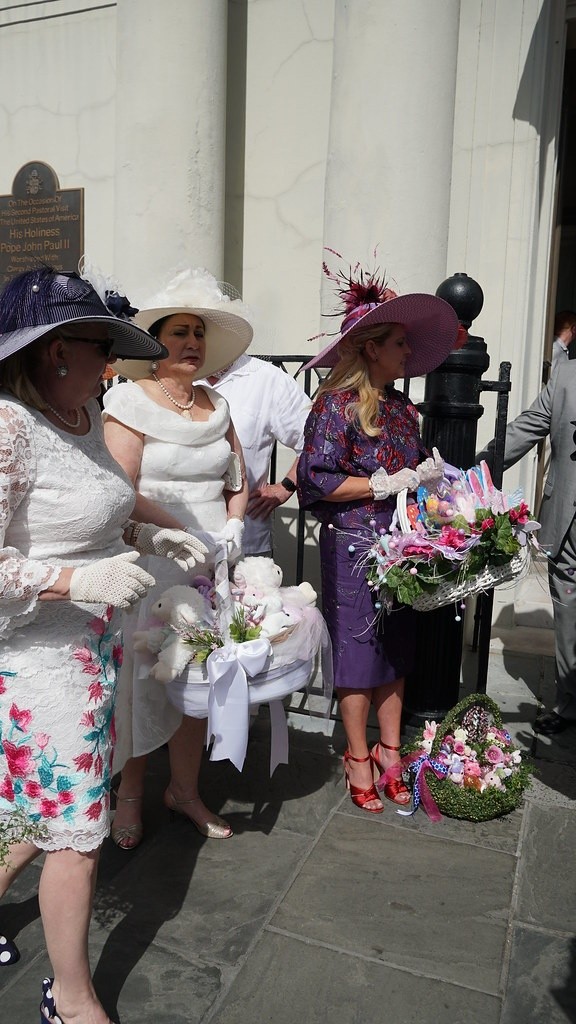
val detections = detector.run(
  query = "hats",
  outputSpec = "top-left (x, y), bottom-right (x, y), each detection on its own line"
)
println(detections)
top-left (0, 271), bottom-right (167, 361)
top-left (103, 285), bottom-right (254, 384)
top-left (299, 293), bottom-right (463, 378)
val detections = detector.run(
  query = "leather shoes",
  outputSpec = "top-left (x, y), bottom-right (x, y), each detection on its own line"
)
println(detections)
top-left (532, 710), bottom-right (570, 734)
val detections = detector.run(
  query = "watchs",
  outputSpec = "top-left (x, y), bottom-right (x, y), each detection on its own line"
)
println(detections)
top-left (281, 477), bottom-right (297, 492)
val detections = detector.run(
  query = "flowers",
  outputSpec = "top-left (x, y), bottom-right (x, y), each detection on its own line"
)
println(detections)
top-left (103, 252), bottom-right (254, 322)
top-left (422, 727), bottom-right (506, 764)
top-left (326, 492), bottom-right (576, 640)
top-left (170, 603), bottom-right (263, 663)
top-left (308, 242), bottom-right (400, 341)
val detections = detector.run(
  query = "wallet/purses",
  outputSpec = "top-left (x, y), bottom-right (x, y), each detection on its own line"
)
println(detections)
top-left (221, 451), bottom-right (243, 493)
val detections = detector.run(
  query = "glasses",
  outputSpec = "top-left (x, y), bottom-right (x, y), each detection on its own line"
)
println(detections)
top-left (60, 335), bottom-right (116, 358)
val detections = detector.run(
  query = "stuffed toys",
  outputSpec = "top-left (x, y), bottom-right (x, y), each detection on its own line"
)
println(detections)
top-left (131, 556), bottom-right (318, 685)
top-left (419, 721), bottom-right (522, 793)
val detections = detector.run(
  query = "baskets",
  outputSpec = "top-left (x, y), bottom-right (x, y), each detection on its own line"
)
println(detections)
top-left (160, 537), bottom-right (314, 716)
top-left (395, 483), bottom-right (528, 609)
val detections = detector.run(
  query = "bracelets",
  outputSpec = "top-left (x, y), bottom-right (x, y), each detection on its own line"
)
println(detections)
top-left (369, 477), bottom-right (373, 497)
top-left (126, 522), bottom-right (145, 547)
top-left (183, 526), bottom-right (187, 532)
top-left (230, 514), bottom-right (244, 522)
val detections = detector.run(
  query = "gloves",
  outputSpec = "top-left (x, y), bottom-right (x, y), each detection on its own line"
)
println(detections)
top-left (185, 518), bottom-right (243, 566)
top-left (137, 525), bottom-right (207, 574)
top-left (68, 549), bottom-right (155, 614)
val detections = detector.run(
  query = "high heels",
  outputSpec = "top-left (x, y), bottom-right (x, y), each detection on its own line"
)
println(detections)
top-left (112, 791), bottom-right (142, 848)
top-left (372, 738), bottom-right (410, 805)
top-left (342, 750), bottom-right (383, 813)
top-left (164, 787), bottom-right (232, 838)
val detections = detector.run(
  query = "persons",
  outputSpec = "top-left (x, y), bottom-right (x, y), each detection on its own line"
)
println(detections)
top-left (552, 309), bottom-right (576, 376)
top-left (298, 282), bottom-right (458, 813)
top-left (192, 355), bottom-right (314, 557)
top-left (101, 269), bottom-right (250, 850)
top-left (473, 359), bottom-right (576, 734)
top-left (0, 268), bottom-right (209, 1024)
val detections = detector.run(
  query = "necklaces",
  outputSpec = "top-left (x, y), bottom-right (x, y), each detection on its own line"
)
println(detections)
top-left (153, 373), bottom-right (195, 409)
top-left (44, 400), bottom-right (81, 428)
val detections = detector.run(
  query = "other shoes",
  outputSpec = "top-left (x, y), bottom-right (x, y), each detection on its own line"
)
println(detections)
top-left (39, 975), bottom-right (69, 1024)
top-left (0, 934), bottom-right (20, 966)
